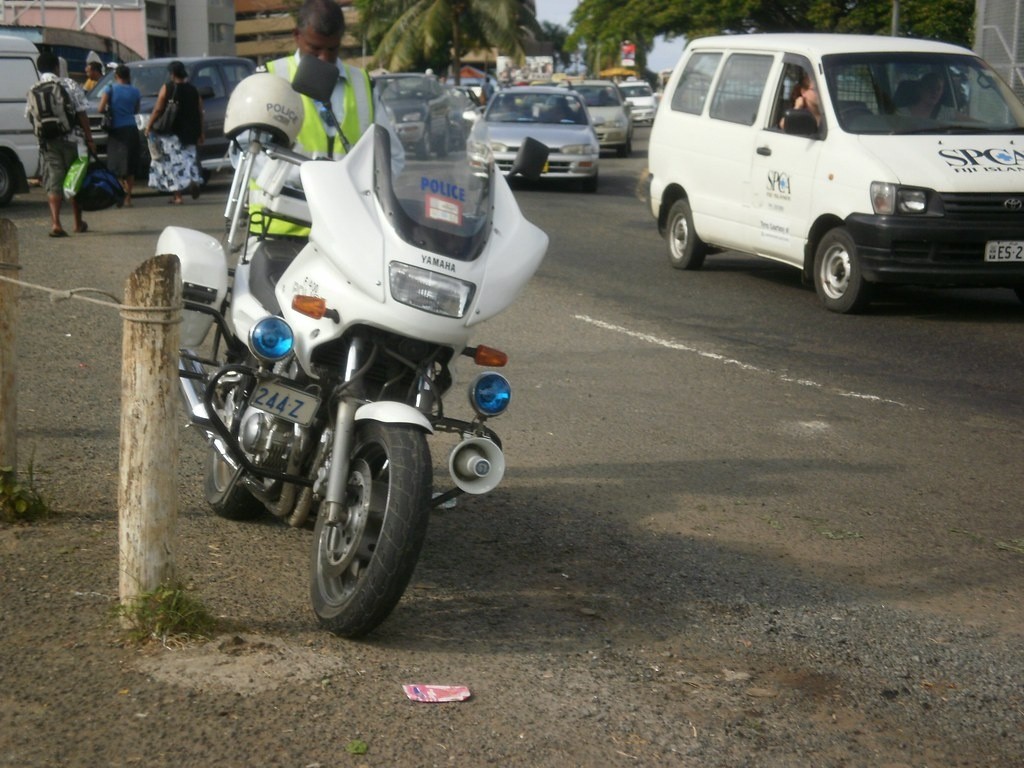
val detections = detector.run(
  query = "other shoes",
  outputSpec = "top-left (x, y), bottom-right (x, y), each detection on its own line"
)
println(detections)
top-left (190, 183), bottom-right (199, 200)
top-left (176, 199), bottom-right (183, 204)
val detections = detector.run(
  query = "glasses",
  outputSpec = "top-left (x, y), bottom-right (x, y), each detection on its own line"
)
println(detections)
top-left (808, 86), bottom-right (818, 93)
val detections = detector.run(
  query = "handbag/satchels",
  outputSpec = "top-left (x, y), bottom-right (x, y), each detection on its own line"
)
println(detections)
top-left (63, 143), bottom-right (89, 200)
top-left (75, 155), bottom-right (128, 210)
top-left (101, 85), bottom-right (114, 132)
top-left (151, 83), bottom-right (179, 132)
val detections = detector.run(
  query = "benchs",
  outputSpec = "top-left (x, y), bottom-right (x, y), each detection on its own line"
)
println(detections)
top-left (718, 99), bottom-right (875, 124)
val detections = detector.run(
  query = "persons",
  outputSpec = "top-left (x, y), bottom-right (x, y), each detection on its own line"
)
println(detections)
top-left (228, 0), bottom-right (405, 246)
top-left (83, 61), bottom-right (105, 95)
top-left (498, 63), bottom-right (512, 82)
top-left (894, 72), bottom-right (991, 123)
top-left (776, 75), bottom-right (821, 133)
top-left (482, 77), bottom-right (494, 102)
top-left (98, 65), bottom-right (141, 207)
top-left (23, 53), bottom-right (96, 237)
top-left (501, 97), bottom-right (525, 116)
top-left (144, 61), bottom-right (205, 204)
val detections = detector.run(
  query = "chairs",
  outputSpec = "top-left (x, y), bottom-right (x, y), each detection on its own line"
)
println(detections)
top-left (894, 80), bottom-right (922, 108)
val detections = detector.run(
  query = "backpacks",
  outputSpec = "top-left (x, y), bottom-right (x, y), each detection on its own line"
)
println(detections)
top-left (28, 77), bottom-right (76, 138)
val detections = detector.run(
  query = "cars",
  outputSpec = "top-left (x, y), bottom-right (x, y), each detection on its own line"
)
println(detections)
top-left (617, 82), bottom-right (659, 123)
top-left (369, 73), bottom-right (453, 160)
top-left (87, 56), bottom-right (259, 194)
top-left (442, 86), bottom-right (483, 143)
top-left (462, 86), bottom-right (600, 193)
top-left (557, 80), bottom-right (634, 158)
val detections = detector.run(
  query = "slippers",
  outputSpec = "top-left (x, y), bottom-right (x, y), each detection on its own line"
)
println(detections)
top-left (49, 228), bottom-right (68, 237)
top-left (73, 221), bottom-right (87, 231)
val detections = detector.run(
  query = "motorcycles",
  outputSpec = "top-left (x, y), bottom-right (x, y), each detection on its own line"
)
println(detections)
top-left (155, 78), bottom-right (551, 638)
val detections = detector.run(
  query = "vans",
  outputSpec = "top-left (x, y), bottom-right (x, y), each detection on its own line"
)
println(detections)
top-left (0, 35), bottom-right (47, 207)
top-left (648, 33), bottom-right (1024, 314)
